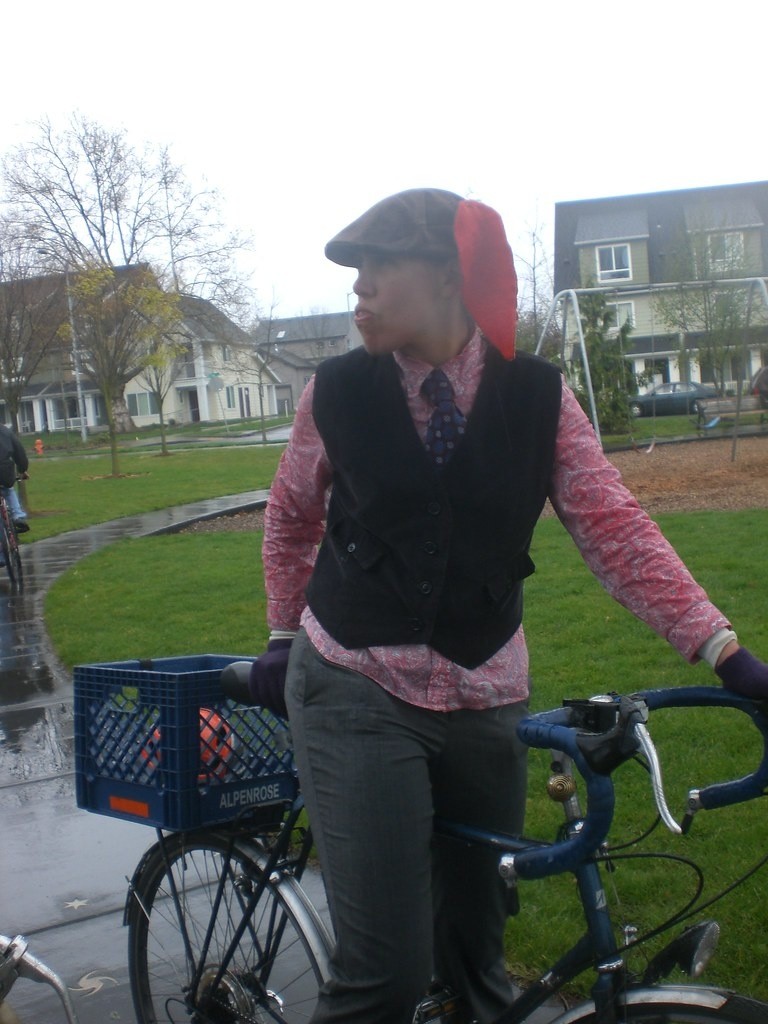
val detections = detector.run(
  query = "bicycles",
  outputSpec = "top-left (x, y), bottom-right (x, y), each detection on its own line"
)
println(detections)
top-left (118, 684), bottom-right (767, 1024)
top-left (0, 474), bottom-right (31, 581)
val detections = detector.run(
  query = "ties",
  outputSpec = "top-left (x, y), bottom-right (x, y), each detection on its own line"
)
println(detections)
top-left (421, 370), bottom-right (464, 465)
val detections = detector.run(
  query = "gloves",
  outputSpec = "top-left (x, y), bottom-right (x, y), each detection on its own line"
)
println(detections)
top-left (250, 639), bottom-right (293, 722)
top-left (715, 647), bottom-right (768, 708)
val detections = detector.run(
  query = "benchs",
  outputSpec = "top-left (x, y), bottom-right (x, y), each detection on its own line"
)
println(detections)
top-left (695, 394), bottom-right (768, 431)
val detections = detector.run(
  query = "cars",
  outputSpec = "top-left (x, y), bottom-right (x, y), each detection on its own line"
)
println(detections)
top-left (630, 380), bottom-right (734, 416)
top-left (750, 366), bottom-right (768, 409)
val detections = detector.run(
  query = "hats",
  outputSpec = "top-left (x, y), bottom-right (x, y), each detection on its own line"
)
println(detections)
top-left (325, 189), bottom-right (517, 360)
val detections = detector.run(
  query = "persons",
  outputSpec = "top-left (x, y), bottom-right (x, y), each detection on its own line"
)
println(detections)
top-left (0, 420), bottom-right (30, 532)
top-left (249, 187), bottom-right (767, 1024)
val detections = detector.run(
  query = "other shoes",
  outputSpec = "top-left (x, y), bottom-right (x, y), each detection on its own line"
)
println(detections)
top-left (13, 518), bottom-right (30, 531)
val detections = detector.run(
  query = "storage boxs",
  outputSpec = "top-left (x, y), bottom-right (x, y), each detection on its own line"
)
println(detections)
top-left (73, 653), bottom-right (300, 835)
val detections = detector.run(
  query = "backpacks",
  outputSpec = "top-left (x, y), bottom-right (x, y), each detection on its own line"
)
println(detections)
top-left (0, 431), bottom-right (15, 487)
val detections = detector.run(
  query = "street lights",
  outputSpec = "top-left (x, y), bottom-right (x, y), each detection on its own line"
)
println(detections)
top-left (36, 249), bottom-right (90, 449)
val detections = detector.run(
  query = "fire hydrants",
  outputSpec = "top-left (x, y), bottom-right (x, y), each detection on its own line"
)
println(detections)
top-left (34, 440), bottom-right (45, 455)
